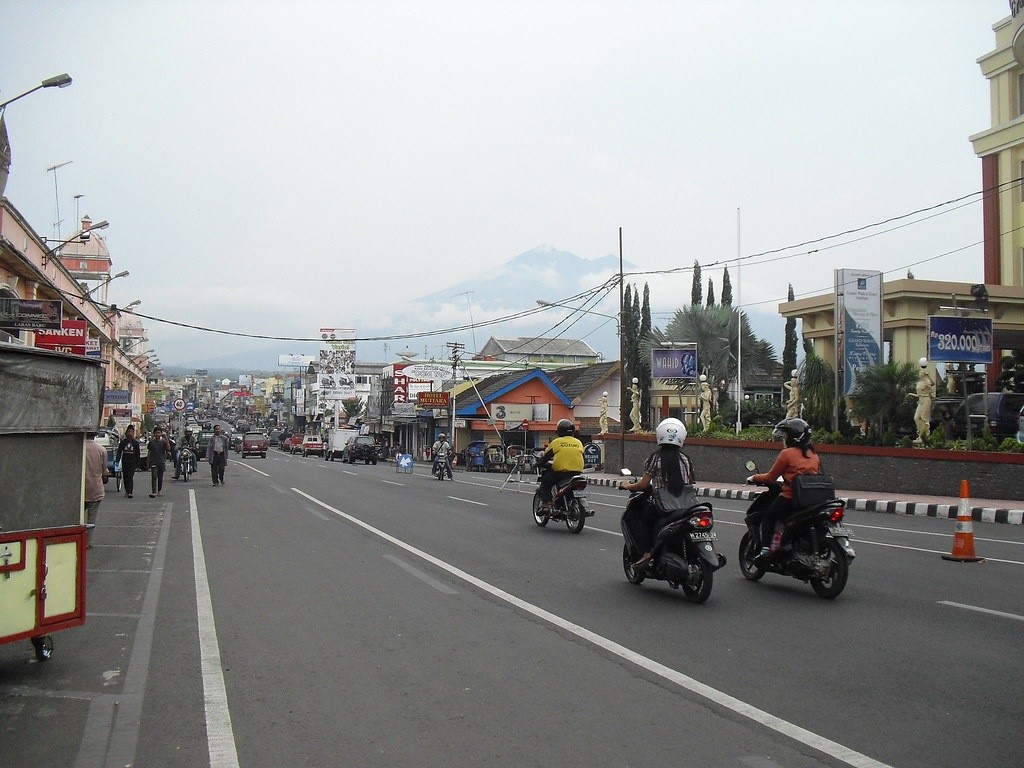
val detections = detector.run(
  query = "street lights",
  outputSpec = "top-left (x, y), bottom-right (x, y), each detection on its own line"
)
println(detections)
top-left (536, 299), bottom-right (625, 476)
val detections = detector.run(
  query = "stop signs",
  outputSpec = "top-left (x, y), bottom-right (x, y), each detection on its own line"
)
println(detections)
top-left (522, 418), bottom-right (530, 430)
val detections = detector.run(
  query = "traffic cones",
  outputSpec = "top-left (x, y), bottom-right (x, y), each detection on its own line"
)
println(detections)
top-left (941, 480), bottom-right (986, 563)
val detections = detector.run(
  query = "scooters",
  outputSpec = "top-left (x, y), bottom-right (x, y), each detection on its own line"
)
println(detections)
top-left (374, 441), bottom-right (403, 462)
top-left (617, 467), bottom-right (728, 605)
top-left (738, 459), bottom-right (858, 600)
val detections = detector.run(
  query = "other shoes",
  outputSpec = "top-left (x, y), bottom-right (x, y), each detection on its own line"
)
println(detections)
top-left (172, 476), bottom-right (179, 479)
top-left (149, 494), bottom-right (156, 498)
top-left (128, 494), bottom-right (133, 498)
top-left (221, 481), bottom-right (225, 484)
top-left (157, 491), bottom-right (163, 496)
top-left (212, 482), bottom-right (217, 486)
top-left (752, 550), bottom-right (771, 563)
top-left (630, 555), bottom-right (654, 569)
top-left (535, 506), bottom-right (549, 516)
top-left (193, 469), bottom-right (197, 472)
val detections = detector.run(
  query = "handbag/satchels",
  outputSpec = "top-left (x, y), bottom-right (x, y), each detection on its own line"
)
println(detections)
top-left (655, 485), bottom-right (701, 513)
top-left (431, 454), bottom-right (437, 461)
top-left (791, 474), bottom-right (836, 509)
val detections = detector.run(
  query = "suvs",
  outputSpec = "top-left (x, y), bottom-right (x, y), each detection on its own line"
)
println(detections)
top-left (930, 391), bottom-right (1024, 449)
top-left (341, 435), bottom-right (378, 465)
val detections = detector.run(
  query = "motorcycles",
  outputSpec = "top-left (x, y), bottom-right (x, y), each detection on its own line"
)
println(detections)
top-left (431, 447), bottom-right (452, 481)
top-left (531, 453), bottom-right (596, 535)
top-left (176, 441), bottom-right (201, 483)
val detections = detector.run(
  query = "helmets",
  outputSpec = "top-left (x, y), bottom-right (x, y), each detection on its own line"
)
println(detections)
top-left (772, 417), bottom-right (811, 446)
top-left (184, 427), bottom-right (193, 436)
top-left (655, 417), bottom-right (687, 448)
top-left (557, 419), bottom-right (575, 437)
top-left (438, 433), bottom-right (446, 438)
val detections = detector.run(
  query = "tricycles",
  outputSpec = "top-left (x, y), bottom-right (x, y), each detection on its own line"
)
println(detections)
top-left (94, 429), bottom-right (124, 492)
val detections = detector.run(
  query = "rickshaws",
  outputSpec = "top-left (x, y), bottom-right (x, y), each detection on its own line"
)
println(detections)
top-left (462, 439), bottom-right (549, 474)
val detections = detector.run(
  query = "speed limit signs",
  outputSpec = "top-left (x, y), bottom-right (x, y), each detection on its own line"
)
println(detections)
top-left (174, 398), bottom-right (186, 410)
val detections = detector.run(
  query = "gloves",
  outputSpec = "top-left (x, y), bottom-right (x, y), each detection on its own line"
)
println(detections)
top-left (746, 475), bottom-right (754, 484)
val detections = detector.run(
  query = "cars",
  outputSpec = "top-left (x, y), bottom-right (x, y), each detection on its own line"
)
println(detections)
top-left (281, 438), bottom-right (291, 452)
top-left (135, 405), bottom-right (296, 472)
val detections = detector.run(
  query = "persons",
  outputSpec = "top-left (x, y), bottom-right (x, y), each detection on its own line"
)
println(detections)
top-left (622, 418), bottom-right (693, 566)
top-left (206, 425), bottom-right (228, 486)
top-left (597, 397), bottom-right (609, 433)
top-left (908, 369), bottom-right (935, 443)
top-left (431, 433), bottom-right (455, 481)
top-left (84, 433), bottom-right (108, 550)
top-left (746, 418), bottom-right (820, 563)
top-left (172, 426), bottom-right (198, 479)
top-left (783, 378), bottom-right (799, 418)
top-left (699, 382), bottom-right (712, 432)
top-left (146, 427), bottom-right (168, 497)
top-left (534, 419), bottom-right (584, 516)
top-left (627, 384), bottom-right (641, 431)
top-left (116, 425), bottom-right (140, 498)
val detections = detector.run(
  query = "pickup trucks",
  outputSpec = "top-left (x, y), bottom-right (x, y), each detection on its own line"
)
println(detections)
top-left (300, 434), bottom-right (324, 457)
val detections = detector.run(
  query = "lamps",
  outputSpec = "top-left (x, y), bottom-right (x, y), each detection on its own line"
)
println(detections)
top-left (128, 349), bottom-right (155, 366)
top-left (80, 270), bottom-right (129, 307)
top-left (0, 73), bottom-right (72, 108)
top-left (119, 338), bottom-right (149, 360)
top-left (138, 358), bottom-right (163, 377)
top-left (103, 300), bottom-right (141, 328)
top-left (133, 354), bottom-right (156, 371)
top-left (42, 221), bottom-right (109, 269)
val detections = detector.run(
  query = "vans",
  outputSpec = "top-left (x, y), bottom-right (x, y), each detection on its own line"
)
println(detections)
top-left (289, 433), bottom-right (306, 455)
top-left (240, 431), bottom-right (269, 459)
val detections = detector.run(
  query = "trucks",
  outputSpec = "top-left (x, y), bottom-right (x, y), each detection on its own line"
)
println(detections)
top-left (325, 428), bottom-right (359, 461)
